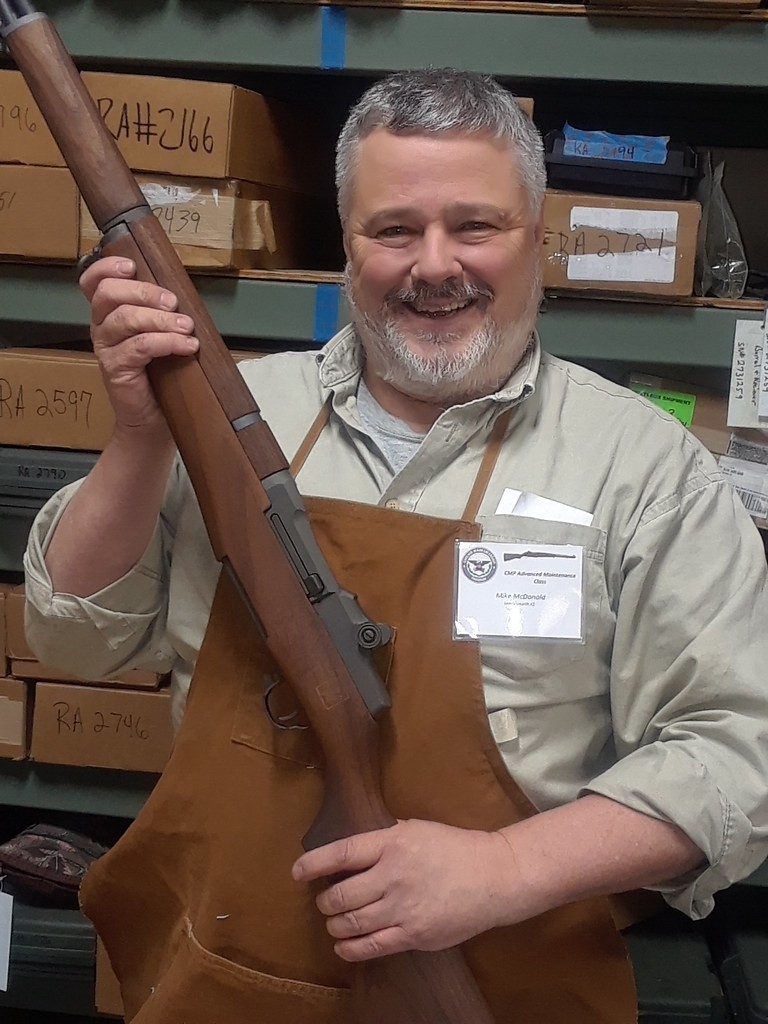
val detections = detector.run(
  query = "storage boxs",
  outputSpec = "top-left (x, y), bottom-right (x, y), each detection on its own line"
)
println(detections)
top-left (1, 675), bottom-right (32, 761)
top-left (0, 346), bottom-right (115, 453)
top-left (7, 581), bottom-right (163, 691)
top-left (80, 171), bottom-right (295, 272)
top-left (542, 190), bottom-right (701, 295)
top-left (624, 371), bottom-right (768, 530)
top-left (1, 164), bottom-right (80, 267)
top-left (0, 69), bottom-right (68, 168)
top-left (31, 681), bottom-right (175, 773)
top-left (80, 70), bottom-right (330, 190)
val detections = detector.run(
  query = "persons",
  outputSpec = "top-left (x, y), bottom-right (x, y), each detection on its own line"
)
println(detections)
top-left (21, 66), bottom-right (768, 1024)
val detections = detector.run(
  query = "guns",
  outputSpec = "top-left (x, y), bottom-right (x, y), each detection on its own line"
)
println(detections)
top-left (1, 0), bottom-right (496, 1024)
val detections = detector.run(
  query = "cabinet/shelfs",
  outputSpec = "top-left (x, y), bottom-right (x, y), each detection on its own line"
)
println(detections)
top-left (0, 0), bottom-right (768, 886)
